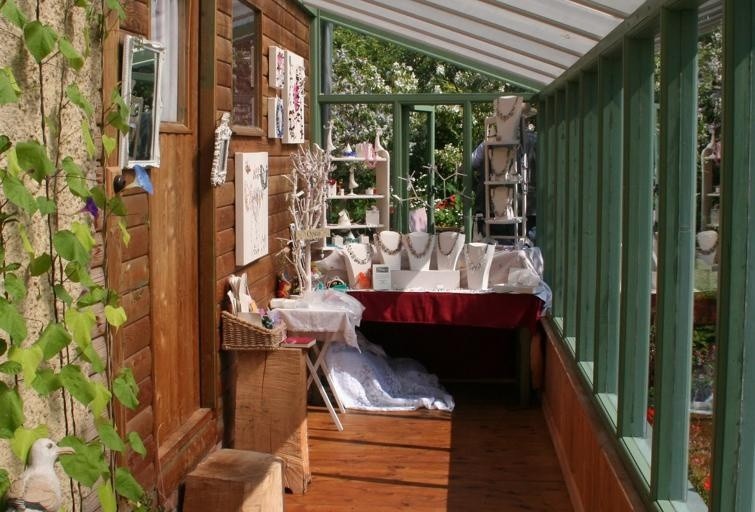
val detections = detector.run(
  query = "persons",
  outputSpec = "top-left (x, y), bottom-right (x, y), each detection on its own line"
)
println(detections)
top-left (489, 186), bottom-right (515, 220)
top-left (371, 230), bottom-right (404, 270)
top-left (487, 145), bottom-right (514, 181)
top-left (435, 230), bottom-right (466, 271)
top-left (491, 95), bottom-right (524, 140)
top-left (404, 232), bottom-right (435, 271)
top-left (340, 243), bottom-right (374, 290)
top-left (463, 242), bottom-right (496, 291)
top-left (693, 230), bottom-right (720, 270)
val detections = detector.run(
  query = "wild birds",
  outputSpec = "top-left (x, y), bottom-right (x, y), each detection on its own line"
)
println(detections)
top-left (1, 436), bottom-right (74, 512)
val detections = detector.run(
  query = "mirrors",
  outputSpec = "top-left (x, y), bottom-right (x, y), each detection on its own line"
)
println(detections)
top-left (117, 32), bottom-right (166, 171)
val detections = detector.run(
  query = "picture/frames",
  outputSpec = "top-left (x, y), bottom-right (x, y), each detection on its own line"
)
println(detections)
top-left (209, 112), bottom-right (233, 186)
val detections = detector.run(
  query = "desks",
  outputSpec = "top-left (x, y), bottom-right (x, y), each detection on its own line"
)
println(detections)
top-left (650, 288), bottom-right (718, 332)
top-left (339, 284), bottom-right (547, 411)
top-left (222, 346), bottom-right (313, 497)
top-left (270, 306), bottom-right (363, 434)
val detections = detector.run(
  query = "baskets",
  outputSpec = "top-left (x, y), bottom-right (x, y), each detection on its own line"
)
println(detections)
top-left (220, 310), bottom-right (286, 352)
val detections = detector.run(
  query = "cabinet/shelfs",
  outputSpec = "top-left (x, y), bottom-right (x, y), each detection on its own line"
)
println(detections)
top-left (484, 116), bottom-right (529, 247)
top-left (700, 143), bottom-right (720, 233)
top-left (311, 141), bottom-right (391, 252)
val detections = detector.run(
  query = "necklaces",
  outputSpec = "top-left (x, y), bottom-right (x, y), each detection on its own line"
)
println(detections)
top-left (465, 243), bottom-right (488, 272)
top-left (490, 189), bottom-right (512, 218)
top-left (696, 237), bottom-right (720, 256)
top-left (496, 95), bottom-right (519, 122)
top-left (345, 243), bottom-right (372, 266)
top-left (404, 233), bottom-right (433, 259)
top-left (437, 231), bottom-right (460, 257)
top-left (375, 231), bottom-right (403, 255)
top-left (489, 147), bottom-right (512, 177)
